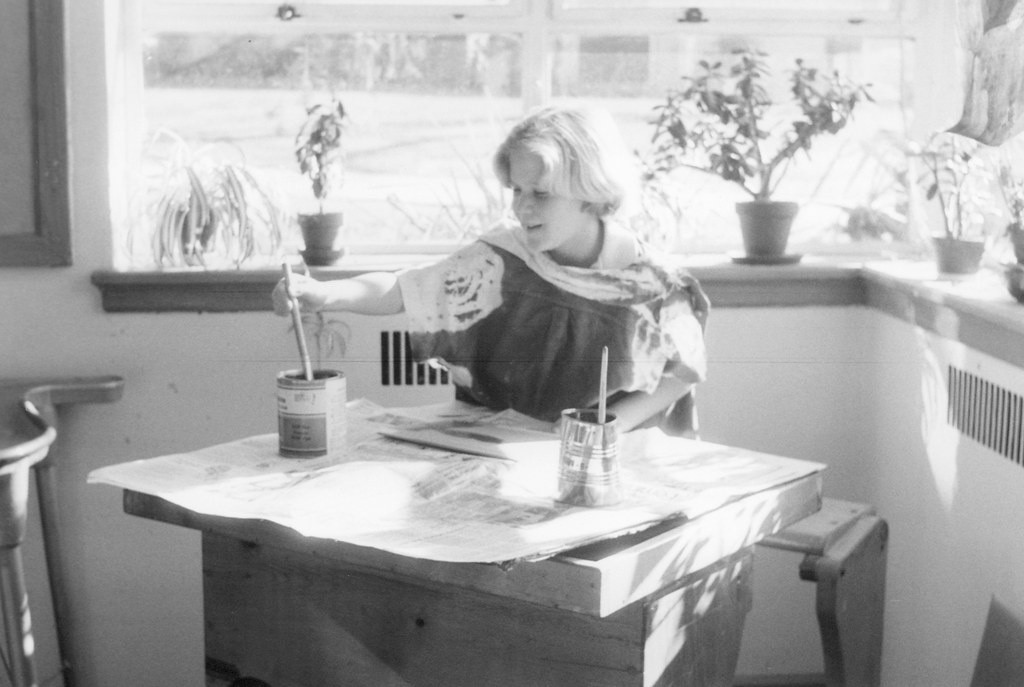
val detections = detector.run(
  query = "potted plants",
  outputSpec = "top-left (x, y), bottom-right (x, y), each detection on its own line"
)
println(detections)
top-left (999, 186), bottom-right (1024, 306)
top-left (628, 44), bottom-right (877, 265)
top-left (904, 130), bottom-right (987, 277)
top-left (122, 129), bottom-right (286, 271)
top-left (292, 100), bottom-right (351, 269)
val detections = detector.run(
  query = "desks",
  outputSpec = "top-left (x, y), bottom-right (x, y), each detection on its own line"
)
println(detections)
top-left (123, 399), bottom-right (826, 687)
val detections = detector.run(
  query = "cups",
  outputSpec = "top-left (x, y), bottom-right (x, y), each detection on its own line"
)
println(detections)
top-left (553, 409), bottom-right (622, 506)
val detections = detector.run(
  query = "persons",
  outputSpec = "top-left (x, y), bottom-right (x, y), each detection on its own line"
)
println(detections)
top-left (272, 106), bottom-right (708, 439)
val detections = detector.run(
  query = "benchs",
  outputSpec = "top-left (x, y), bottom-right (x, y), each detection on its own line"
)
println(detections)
top-left (752, 499), bottom-right (892, 687)
